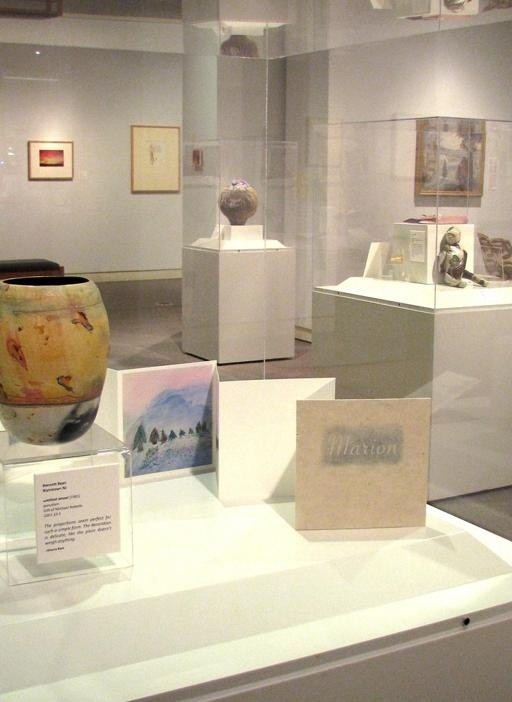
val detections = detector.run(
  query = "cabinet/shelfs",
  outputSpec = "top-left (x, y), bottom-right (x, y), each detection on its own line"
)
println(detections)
top-left (0, 277), bottom-right (512, 702)
top-left (179, 139), bottom-right (298, 366)
top-left (180, 2), bottom-right (298, 61)
top-left (310, 116), bottom-right (512, 503)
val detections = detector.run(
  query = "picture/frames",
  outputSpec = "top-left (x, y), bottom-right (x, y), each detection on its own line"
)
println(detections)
top-left (130, 123), bottom-right (183, 194)
top-left (27, 140), bottom-right (76, 182)
top-left (414, 117), bottom-right (488, 198)
